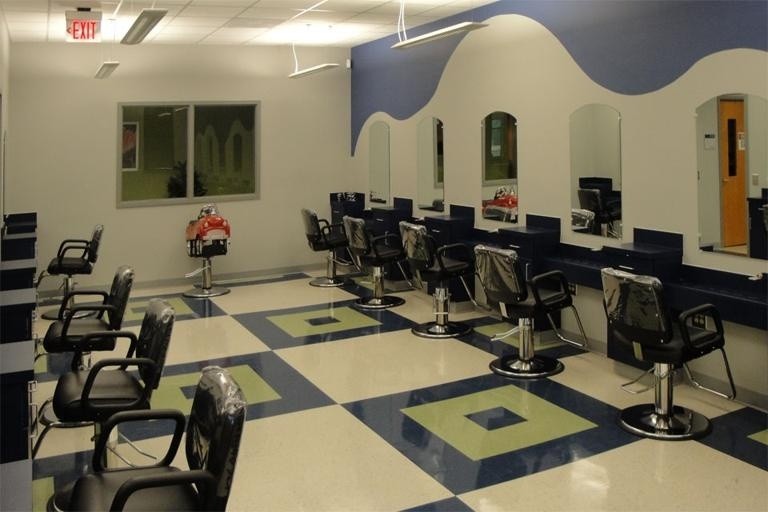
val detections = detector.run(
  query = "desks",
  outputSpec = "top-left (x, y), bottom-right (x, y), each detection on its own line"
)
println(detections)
top-left (1, 212), bottom-right (38, 512)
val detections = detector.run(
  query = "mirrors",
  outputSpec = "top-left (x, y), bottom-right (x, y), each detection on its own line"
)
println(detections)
top-left (367, 119), bottom-right (391, 206)
top-left (413, 116), bottom-right (444, 213)
top-left (481, 109), bottom-right (518, 223)
top-left (568, 102), bottom-right (621, 239)
top-left (694, 90), bottom-right (766, 263)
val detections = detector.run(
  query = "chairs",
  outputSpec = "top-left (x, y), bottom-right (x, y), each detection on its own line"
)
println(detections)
top-left (397, 220), bottom-right (473, 340)
top-left (596, 265), bottom-right (736, 440)
top-left (33, 298), bottom-right (175, 473)
top-left (38, 365), bottom-right (246, 511)
top-left (471, 243), bottom-right (591, 379)
top-left (301, 207), bottom-right (353, 288)
top-left (577, 173), bottom-right (621, 237)
top-left (180, 202), bottom-right (231, 299)
top-left (35, 224), bottom-right (104, 319)
top-left (34, 265), bottom-right (134, 371)
top-left (343, 213), bottom-right (411, 309)
